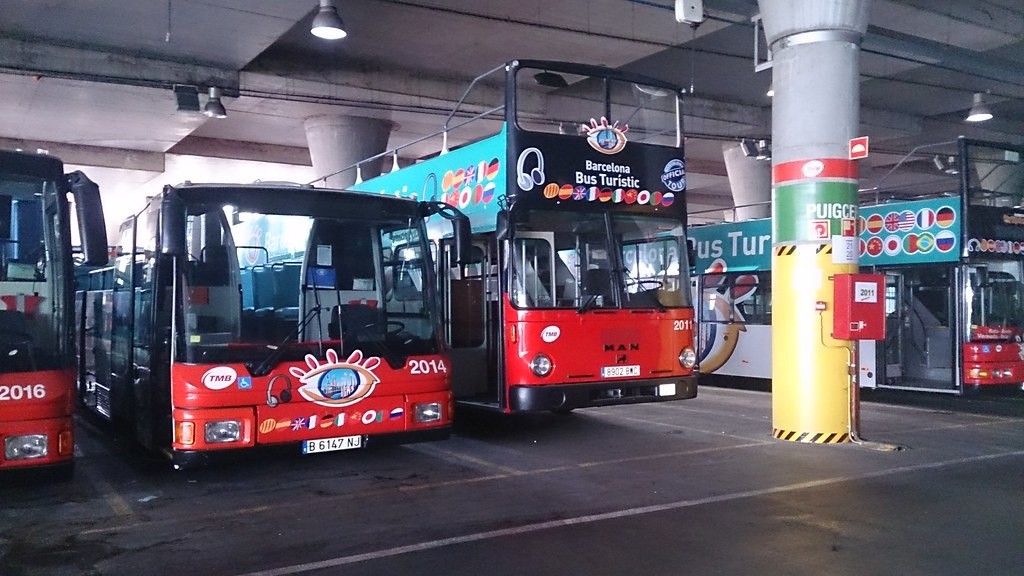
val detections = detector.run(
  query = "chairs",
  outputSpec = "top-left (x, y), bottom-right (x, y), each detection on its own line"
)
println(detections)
top-left (239, 261), bottom-right (302, 329)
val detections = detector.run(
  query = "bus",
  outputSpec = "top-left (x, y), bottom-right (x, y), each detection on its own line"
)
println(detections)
top-left (0, 150), bottom-right (111, 469)
top-left (78, 175), bottom-right (472, 457)
top-left (239, 55), bottom-right (702, 436)
top-left (567, 136), bottom-right (1023, 397)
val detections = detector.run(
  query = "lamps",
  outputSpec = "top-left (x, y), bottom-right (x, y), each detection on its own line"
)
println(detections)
top-left (740, 139), bottom-right (759, 157)
top-left (309, 0), bottom-right (347, 40)
top-left (945, 155), bottom-right (959, 174)
top-left (171, 84), bottom-right (200, 112)
top-left (202, 87), bottom-right (228, 118)
top-left (756, 140), bottom-right (771, 160)
top-left (967, 92), bottom-right (994, 121)
top-left (933, 155), bottom-right (950, 172)
